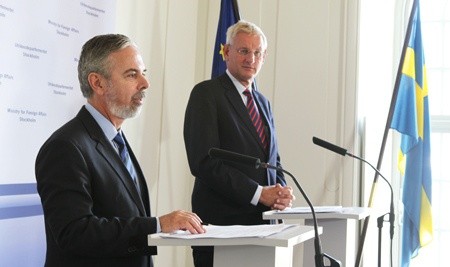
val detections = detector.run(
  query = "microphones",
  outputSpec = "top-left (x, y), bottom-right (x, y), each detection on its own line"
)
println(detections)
top-left (207, 147), bottom-right (322, 255)
top-left (312, 136), bottom-right (394, 223)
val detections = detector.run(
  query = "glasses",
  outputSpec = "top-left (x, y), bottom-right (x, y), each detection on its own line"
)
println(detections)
top-left (234, 47), bottom-right (261, 58)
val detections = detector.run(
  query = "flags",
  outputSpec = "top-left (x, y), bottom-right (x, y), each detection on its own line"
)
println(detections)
top-left (389, 0), bottom-right (434, 267)
top-left (210, 0), bottom-right (256, 90)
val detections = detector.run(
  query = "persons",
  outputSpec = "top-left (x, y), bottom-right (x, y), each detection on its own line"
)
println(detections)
top-left (183, 21), bottom-right (296, 267)
top-left (35, 34), bottom-right (206, 267)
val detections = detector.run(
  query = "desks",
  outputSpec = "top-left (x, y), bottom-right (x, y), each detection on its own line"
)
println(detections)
top-left (263, 205), bottom-right (372, 267)
top-left (147, 225), bottom-right (324, 267)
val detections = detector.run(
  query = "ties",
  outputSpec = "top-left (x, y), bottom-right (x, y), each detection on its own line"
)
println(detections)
top-left (242, 89), bottom-right (269, 154)
top-left (112, 134), bottom-right (142, 195)
